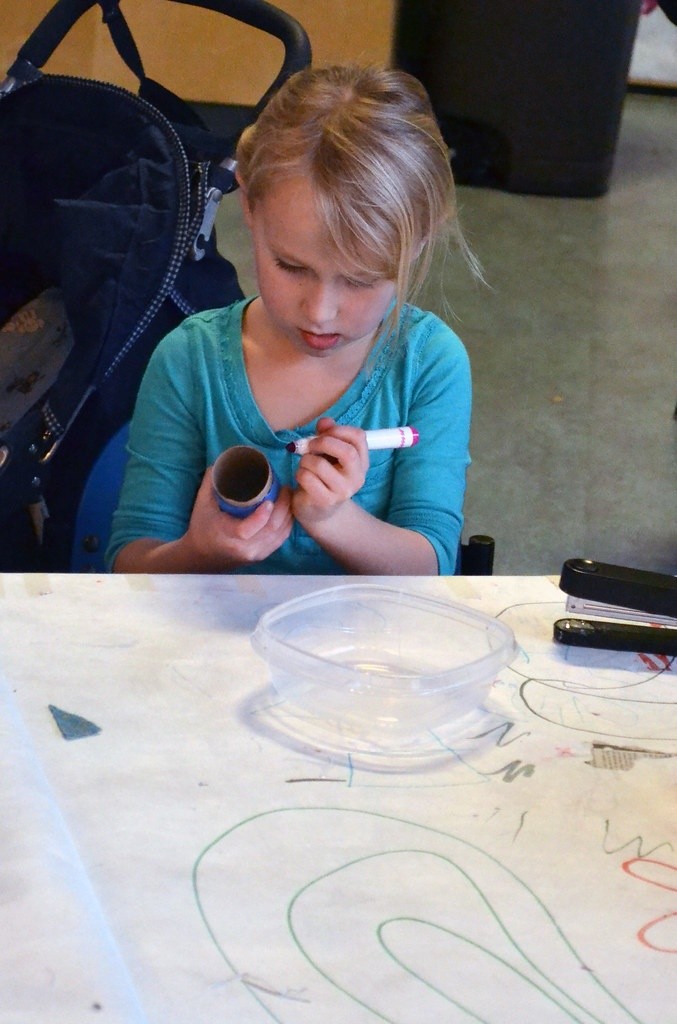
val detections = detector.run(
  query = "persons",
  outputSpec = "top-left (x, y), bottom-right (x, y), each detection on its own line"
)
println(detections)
top-left (102, 60), bottom-right (476, 578)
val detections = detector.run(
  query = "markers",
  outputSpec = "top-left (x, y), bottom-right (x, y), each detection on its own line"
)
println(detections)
top-left (285, 426), bottom-right (420, 456)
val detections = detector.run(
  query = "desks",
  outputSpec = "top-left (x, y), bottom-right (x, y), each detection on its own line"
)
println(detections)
top-left (0, 572), bottom-right (677, 1024)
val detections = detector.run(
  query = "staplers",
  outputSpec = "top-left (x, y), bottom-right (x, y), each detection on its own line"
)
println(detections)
top-left (553, 555), bottom-right (677, 657)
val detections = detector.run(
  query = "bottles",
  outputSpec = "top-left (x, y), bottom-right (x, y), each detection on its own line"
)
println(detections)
top-left (210, 446), bottom-right (283, 518)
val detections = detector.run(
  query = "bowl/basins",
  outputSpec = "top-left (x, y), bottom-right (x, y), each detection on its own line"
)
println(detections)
top-left (250, 583), bottom-right (517, 739)
